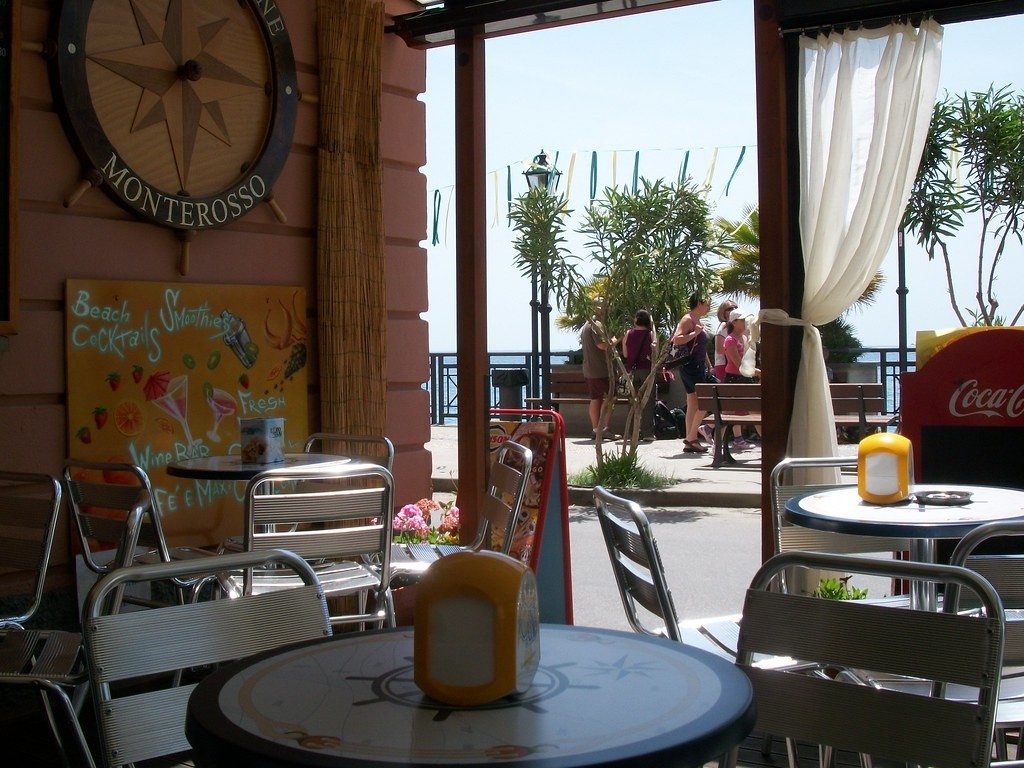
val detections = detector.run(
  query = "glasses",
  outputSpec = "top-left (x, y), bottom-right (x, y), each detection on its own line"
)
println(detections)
top-left (724, 306), bottom-right (738, 312)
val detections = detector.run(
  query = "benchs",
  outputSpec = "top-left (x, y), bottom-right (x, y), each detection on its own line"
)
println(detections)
top-left (696, 382), bottom-right (895, 468)
top-left (526, 373), bottom-right (637, 434)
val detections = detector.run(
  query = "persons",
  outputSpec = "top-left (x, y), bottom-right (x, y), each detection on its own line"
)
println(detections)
top-left (673, 292), bottom-right (833, 452)
top-left (622, 310), bottom-right (658, 441)
top-left (579, 295), bottom-right (623, 440)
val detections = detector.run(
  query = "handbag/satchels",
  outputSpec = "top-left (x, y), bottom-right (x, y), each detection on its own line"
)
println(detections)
top-left (665, 312), bottom-right (698, 369)
top-left (618, 374), bottom-right (634, 396)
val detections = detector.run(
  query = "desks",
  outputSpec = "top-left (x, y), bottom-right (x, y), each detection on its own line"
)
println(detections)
top-left (168, 451), bottom-right (357, 572)
top-left (184, 623), bottom-right (762, 768)
top-left (786, 483), bottom-right (1024, 612)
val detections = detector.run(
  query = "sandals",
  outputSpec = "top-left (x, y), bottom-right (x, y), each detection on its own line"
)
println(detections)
top-left (683, 440), bottom-right (708, 453)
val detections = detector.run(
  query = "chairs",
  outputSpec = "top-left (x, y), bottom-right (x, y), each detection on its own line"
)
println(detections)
top-left (0, 434), bottom-right (1024, 768)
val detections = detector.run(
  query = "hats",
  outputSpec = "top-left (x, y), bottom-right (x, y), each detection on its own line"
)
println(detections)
top-left (729, 308), bottom-right (754, 322)
top-left (594, 297), bottom-right (612, 306)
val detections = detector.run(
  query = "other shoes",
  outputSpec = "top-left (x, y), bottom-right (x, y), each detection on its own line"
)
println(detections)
top-left (698, 425), bottom-right (714, 445)
top-left (591, 430), bottom-right (622, 441)
top-left (733, 441), bottom-right (756, 449)
top-left (638, 434), bottom-right (657, 440)
